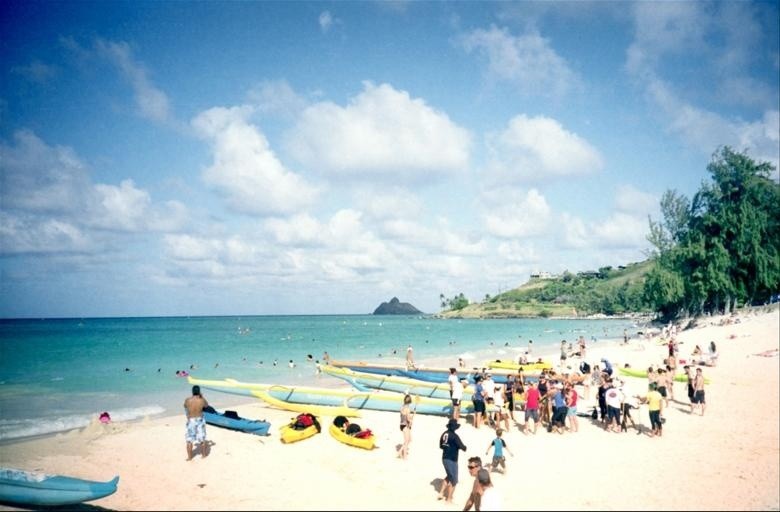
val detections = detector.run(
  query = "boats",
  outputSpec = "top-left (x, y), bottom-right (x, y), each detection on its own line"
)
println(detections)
top-left (185, 405), bottom-right (270, 436)
top-left (186, 375), bottom-right (499, 416)
top-left (0, 466), bottom-right (118, 508)
top-left (279, 413), bottom-right (375, 451)
top-left (320, 359), bottom-right (554, 405)
top-left (623, 367), bottom-right (707, 384)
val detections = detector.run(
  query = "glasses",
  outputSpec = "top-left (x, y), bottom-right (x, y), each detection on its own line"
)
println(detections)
top-left (467, 465), bottom-right (478, 469)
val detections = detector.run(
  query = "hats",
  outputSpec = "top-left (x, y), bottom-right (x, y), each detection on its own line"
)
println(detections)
top-left (446, 419), bottom-right (460, 430)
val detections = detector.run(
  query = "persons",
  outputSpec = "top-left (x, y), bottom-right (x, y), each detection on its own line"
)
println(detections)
top-left (99, 312), bottom-right (744, 512)
